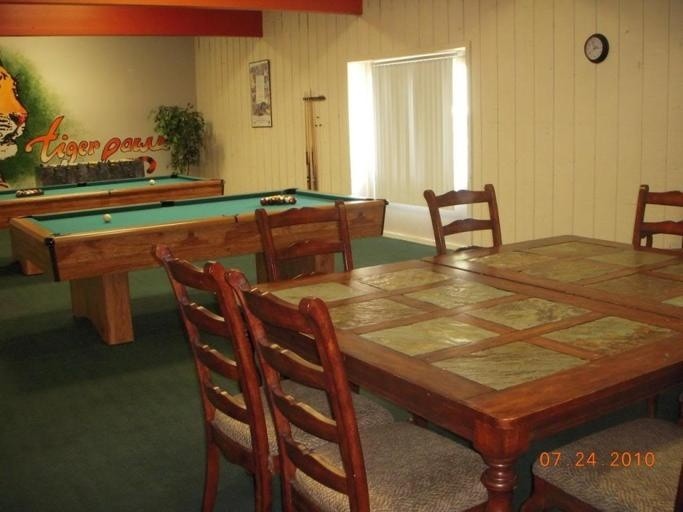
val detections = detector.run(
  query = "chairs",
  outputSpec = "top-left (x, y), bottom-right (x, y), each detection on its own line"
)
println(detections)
top-left (254, 200), bottom-right (353, 283)
top-left (423, 184), bottom-right (502, 255)
top-left (151, 242), bottom-right (395, 512)
top-left (224, 270), bottom-right (490, 512)
top-left (632, 184), bottom-right (683, 251)
top-left (518, 414), bottom-right (683, 511)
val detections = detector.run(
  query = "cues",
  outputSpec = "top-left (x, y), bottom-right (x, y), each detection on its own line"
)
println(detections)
top-left (303, 89), bottom-right (318, 191)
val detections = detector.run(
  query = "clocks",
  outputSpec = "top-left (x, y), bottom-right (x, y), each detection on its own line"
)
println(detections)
top-left (584, 33), bottom-right (609, 64)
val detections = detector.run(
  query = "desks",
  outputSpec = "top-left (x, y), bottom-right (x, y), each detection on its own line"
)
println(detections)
top-left (0, 172), bottom-right (224, 276)
top-left (240, 234), bottom-right (683, 512)
top-left (0, 189), bottom-right (389, 346)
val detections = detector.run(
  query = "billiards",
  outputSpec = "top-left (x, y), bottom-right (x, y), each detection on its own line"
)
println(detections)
top-left (16, 190), bottom-right (43, 195)
top-left (261, 195), bottom-right (296, 205)
top-left (149, 180), bottom-right (155, 185)
top-left (104, 214), bottom-right (111, 222)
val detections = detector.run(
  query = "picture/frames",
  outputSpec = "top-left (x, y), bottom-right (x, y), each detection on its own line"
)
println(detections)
top-left (248, 59), bottom-right (272, 128)
